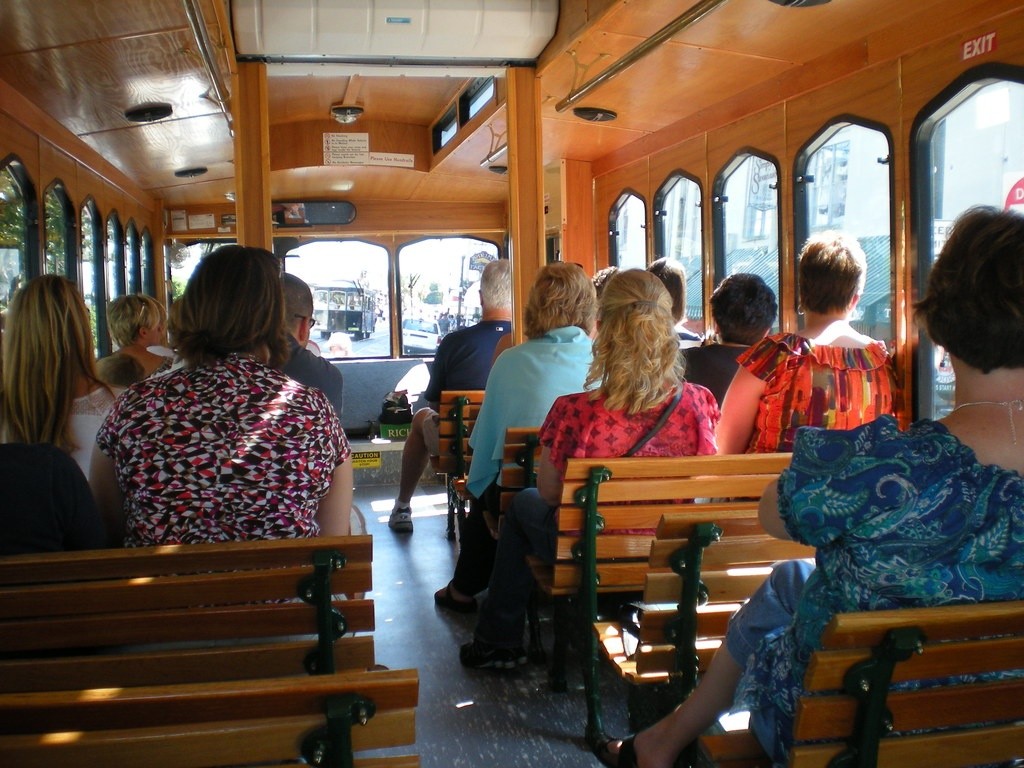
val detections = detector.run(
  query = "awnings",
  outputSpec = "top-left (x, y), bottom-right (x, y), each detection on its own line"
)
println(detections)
top-left (676, 234), bottom-right (891, 324)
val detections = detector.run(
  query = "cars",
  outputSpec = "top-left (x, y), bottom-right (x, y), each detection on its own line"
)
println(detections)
top-left (400, 316), bottom-right (443, 358)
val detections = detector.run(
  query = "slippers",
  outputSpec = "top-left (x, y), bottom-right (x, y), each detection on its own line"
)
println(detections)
top-left (434, 578), bottom-right (478, 614)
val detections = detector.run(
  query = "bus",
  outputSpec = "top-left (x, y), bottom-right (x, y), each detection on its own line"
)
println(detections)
top-left (310, 287), bottom-right (377, 341)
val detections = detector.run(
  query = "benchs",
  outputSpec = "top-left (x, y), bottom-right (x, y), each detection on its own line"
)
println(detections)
top-left (0, 534), bottom-right (374, 694)
top-left (430, 391), bottom-right (485, 540)
top-left (524, 452), bottom-right (792, 693)
top-left (484, 426), bottom-right (540, 540)
top-left (0, 667), bottom-right (421, 768)
top-left (590, 509), bottom-right (816, 728)
top-left (698, 601), bottom-right (1024, 768)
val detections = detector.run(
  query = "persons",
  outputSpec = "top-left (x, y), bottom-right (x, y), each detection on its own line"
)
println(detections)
top-left (95, 351), bottom-right (145, 388)
top-left (585, 206), bottom-right (1024, 768)
top-left (88, 245), bottom-right (390, 672)
top-left (715, 229), bottom-right (905, 503)
top-left (589, 266), bottom-right (620, 340)
top-left (419, 307), bottom-right (482, 337)
top-left (2, 274), bottom-right (129, 483)
top-left (107, 292), bottom-right (189, 381)
top-left (678, 273), bottom-right (778, 410)
top-left (1, 441), bottom-right (109, 661)
top-left (459, 269), bottom-right (721, 672)
top-left (272, 203), bottom-right (309, 225)
top-left (267, 272), bottom-right (343, 419)
top-left (388, 259), bottom-right (513, 533)
top-left (645, 256), bottom-right (701, 341)
top-left (435, 263), bottom-right (609, 614)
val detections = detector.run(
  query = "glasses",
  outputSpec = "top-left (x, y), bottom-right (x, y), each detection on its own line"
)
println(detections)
top-left (295, 314), bottom-right (315, 329)
top-left (127, 294), bottom-right (149, 338)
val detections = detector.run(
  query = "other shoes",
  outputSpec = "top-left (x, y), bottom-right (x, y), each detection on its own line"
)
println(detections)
top-left (584, 723), bottom-right (639, 768)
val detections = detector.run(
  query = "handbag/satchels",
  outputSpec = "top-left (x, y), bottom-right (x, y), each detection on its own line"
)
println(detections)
top-left (378, 391), bottom-right (413, 424)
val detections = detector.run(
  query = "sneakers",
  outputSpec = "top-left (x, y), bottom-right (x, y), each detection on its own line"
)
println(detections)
top-left (460, 637), bottom-right (529, 671)
top-left (388, 499), bottom-right (413, 532)
